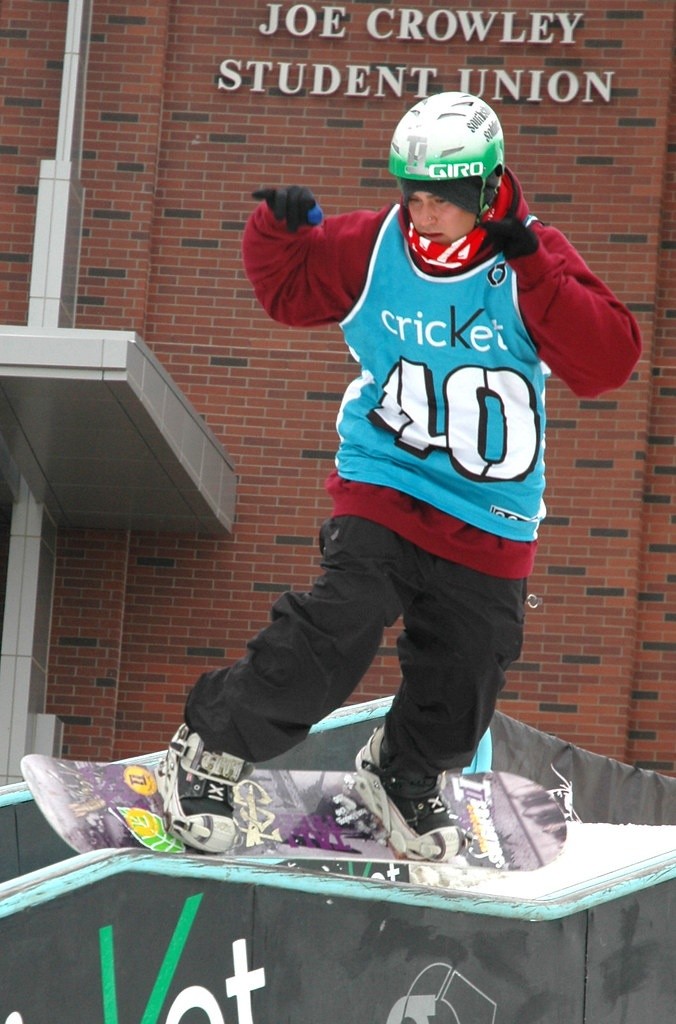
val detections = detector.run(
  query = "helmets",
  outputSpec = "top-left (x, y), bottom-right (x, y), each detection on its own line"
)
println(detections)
top-left (387, 91), bottom-right (506, 181)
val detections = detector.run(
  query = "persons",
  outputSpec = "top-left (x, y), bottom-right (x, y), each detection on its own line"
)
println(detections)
top-left (164, 91), bottom-right (643, 862)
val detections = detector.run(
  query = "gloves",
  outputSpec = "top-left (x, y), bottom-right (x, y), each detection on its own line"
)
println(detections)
top-left (251, 185), bottom-right (324, 232)
top-left (479, 214), bottom-right (537, 260)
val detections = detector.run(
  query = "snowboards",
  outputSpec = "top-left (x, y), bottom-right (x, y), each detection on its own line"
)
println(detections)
top-left (21, 750), bottom-right (567, 874)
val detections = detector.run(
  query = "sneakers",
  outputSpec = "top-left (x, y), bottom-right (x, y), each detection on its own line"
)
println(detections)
top-left (355, 722), bottom-right (460, 861)
top-left (154, 723), bottom-right (245, 851)
